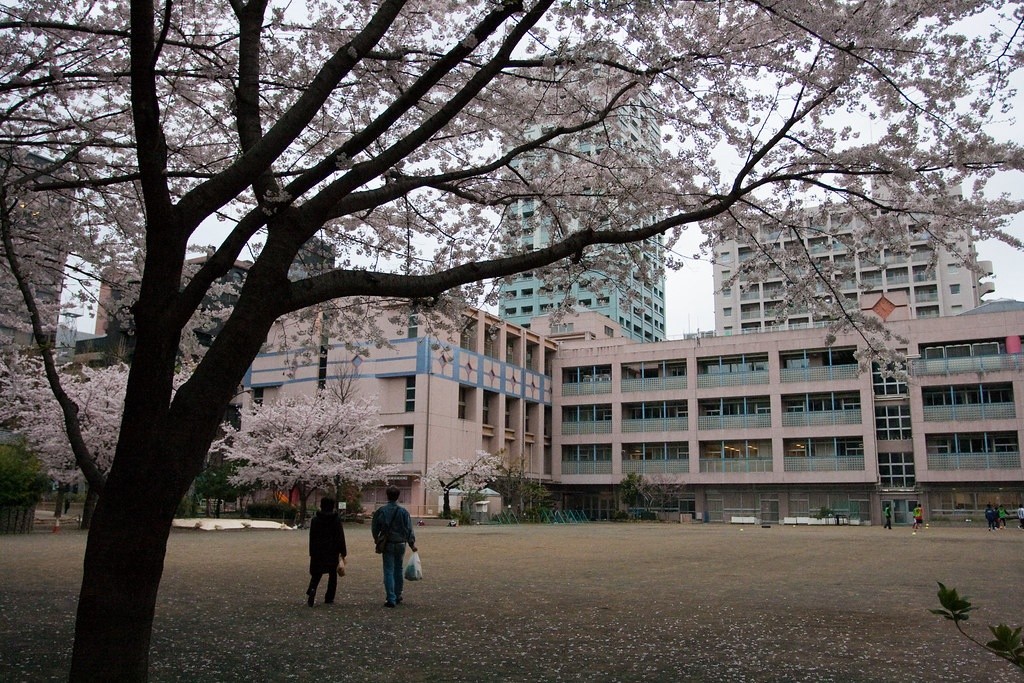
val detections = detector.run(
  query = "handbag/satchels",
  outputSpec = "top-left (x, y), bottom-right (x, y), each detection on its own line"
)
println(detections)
top-left (375, 534), bottom-right (384, 554)
top-left (404, 552), bottom-right (423, 581)
top-left (337, 554), bottom-right (346, 576)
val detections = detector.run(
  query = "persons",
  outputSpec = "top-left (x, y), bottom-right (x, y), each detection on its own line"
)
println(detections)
top-left (305, 496), bottom-right (349, 607)
top-left (910, 503), bottom-right (930, 535)
top-left (993, 503), bottom-right (1010, 531)
top-left (883, 503), bottom-right (892, 530)
top-left (371, 485), bottom-right (419, 608)
top-left (984, 503), bottom-right (997, 531)
top-left (1016, 503), bottom-right (1024, 532)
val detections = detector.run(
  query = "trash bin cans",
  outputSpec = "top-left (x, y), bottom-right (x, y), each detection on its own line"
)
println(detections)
top-left (837, 515), bottom-right (848, 525)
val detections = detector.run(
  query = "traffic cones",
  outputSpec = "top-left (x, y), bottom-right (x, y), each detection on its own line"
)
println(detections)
top-left (51, 517), bottom-right (61, 534)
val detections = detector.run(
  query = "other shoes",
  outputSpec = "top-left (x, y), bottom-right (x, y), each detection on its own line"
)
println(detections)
top-left (988, 526), bottom-right (1006, 531)
top-left (385, 601), bottom-right (395, 607)
top-left (396, 596), bottom-right (403, 603)
top-left (325, 599), bottom-right (334, 603)
top-left (308, 588), bottom-right (315, 607)
top-left (1018, 526), bottom-right (1024, 530)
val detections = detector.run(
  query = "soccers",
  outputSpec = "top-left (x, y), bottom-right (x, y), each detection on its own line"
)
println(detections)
top-left (925, 523), bottom-right (930, 527)
